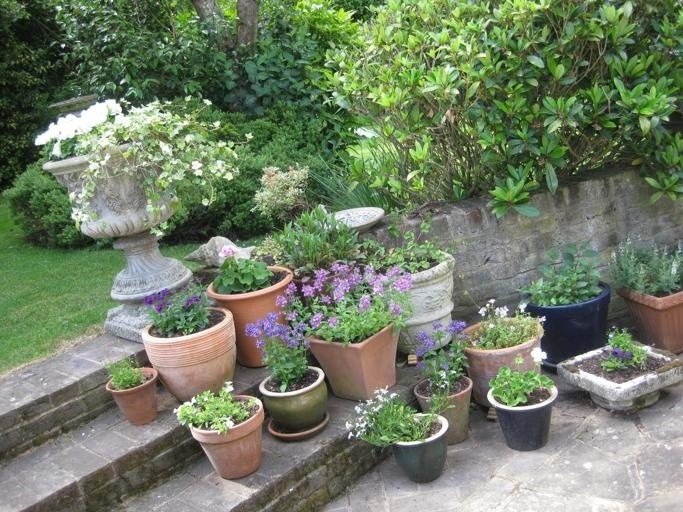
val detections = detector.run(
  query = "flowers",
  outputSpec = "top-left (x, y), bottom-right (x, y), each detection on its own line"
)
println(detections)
top-left (604, 325), bottom-right (646, 373)
top-left (33, 98), bottom-right (253, 238)
top-left (252, 162), bottom-right (309, 218)
top-left (276, 263), bottom-right (412, 343)
top-left (343, 378), bottom-right (453, 447)
top-left (471, 296), bottom-right (546, 347)
top-left (609, 231), bottom-right (683, 294)
top-left (410, 320), bottom-right (468, 394)
top-left (213, 243), bottom-right (274, 293)
top-left (244, 310), bottom-right (311, 390)
top-left (142, 278), bottom-right (210, 334)
top-left (488, 346), bottom-right (554, 407)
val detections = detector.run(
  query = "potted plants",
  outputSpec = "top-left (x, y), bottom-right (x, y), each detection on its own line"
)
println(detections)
top-left (102, 352), bottom-right (158, 427)
top-left (364, 199), bottom-right (458, 353)
top-left (272, 203), bottom-right (363, 303)
top-left (172, 382), bottom-right (265, 480)
top-left (518, 237), bottom-right (612, 375)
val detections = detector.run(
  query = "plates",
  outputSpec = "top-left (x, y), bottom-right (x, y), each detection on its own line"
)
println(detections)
top-left (319, 207), bottom-right (385, 232)
top-left (267, 414), bottom-right (332, 438)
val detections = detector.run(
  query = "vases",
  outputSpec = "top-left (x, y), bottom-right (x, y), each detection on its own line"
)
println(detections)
top-left (413, 374), bottom-right (472, 446)
top-left (460, 315), bottom-right (546, 409)
top-left (140, 307), bottom-right (237, 402)
top-left (616, 282), bottom-right (683, 356)
top-left (206, 263), bottom-right (295, 369)
top-left (259, 366), bottom-right (331, 441)
top-left (392, 413), bottom-right (451, 485)
top-left (558, 337), bottom-right (683, 411)
top-left (40, 142), bottom-right (200, 346)
top-left (485, 380), bottom-right (559, 452)
top-left (305, 318), bottom-right (403, 403)
